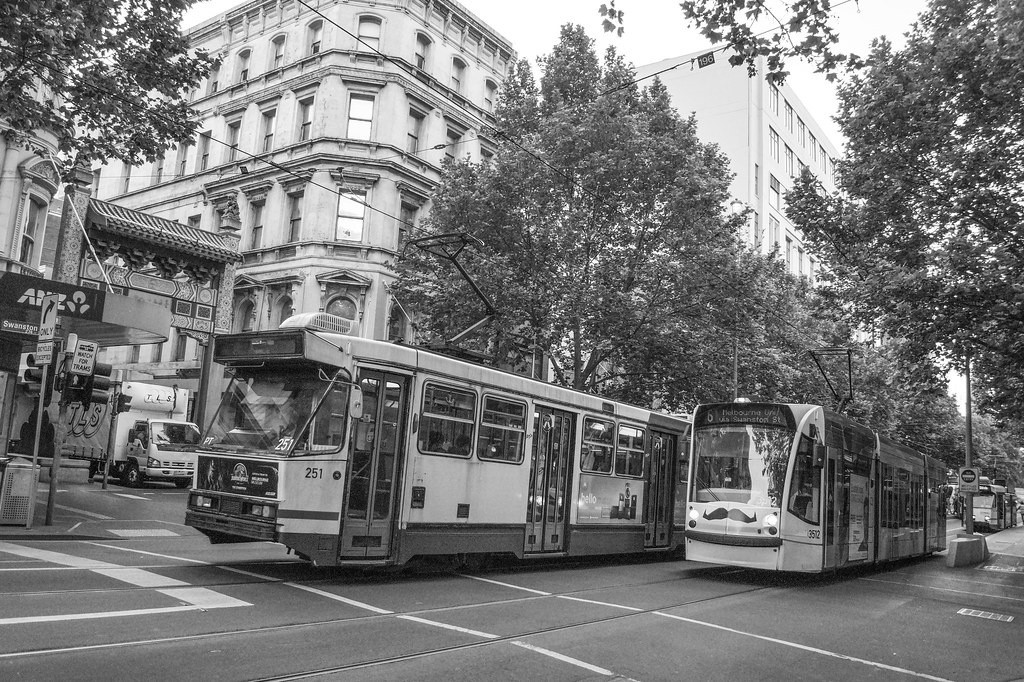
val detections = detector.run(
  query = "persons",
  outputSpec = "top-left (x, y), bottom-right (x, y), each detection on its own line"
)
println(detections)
top-left (422, 429), bottom-right (513, 459)
top-left (946, 495), bottom-right (962, 515)
top-left (1016, 501), bottom-right (1024, 526)
top-left (138, 431), bottom-right (148, 448)
top-left (583, 450), bottom-right (633, 475)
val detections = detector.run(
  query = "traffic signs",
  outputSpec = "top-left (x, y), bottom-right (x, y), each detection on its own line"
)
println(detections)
top-left (38, 293), bottom-right (59, 342)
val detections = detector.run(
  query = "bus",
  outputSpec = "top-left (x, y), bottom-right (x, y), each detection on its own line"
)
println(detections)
top-left (183, 230), bottom-right (692, 575)
top-left (678, 349), bottom-right (948, 575)
top-left (963, 477), bottom-right (1017, 533)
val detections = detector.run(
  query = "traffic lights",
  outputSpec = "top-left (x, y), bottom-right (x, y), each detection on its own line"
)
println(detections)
top-left (115, 391), bottom-right (132, 415)
top-left (66, 371), bottom-right (87, 390)
top-left (82, 357), bottom-right (112, 411)
top-left (24, 343), bottom-right (58, 407)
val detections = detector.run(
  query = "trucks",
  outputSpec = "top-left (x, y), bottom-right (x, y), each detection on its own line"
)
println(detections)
top-left (66, 380), bottom-right (203, 489)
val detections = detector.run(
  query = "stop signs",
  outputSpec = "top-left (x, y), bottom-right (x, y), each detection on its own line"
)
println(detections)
top-left (960, 466), bottom-right (978, 493)
